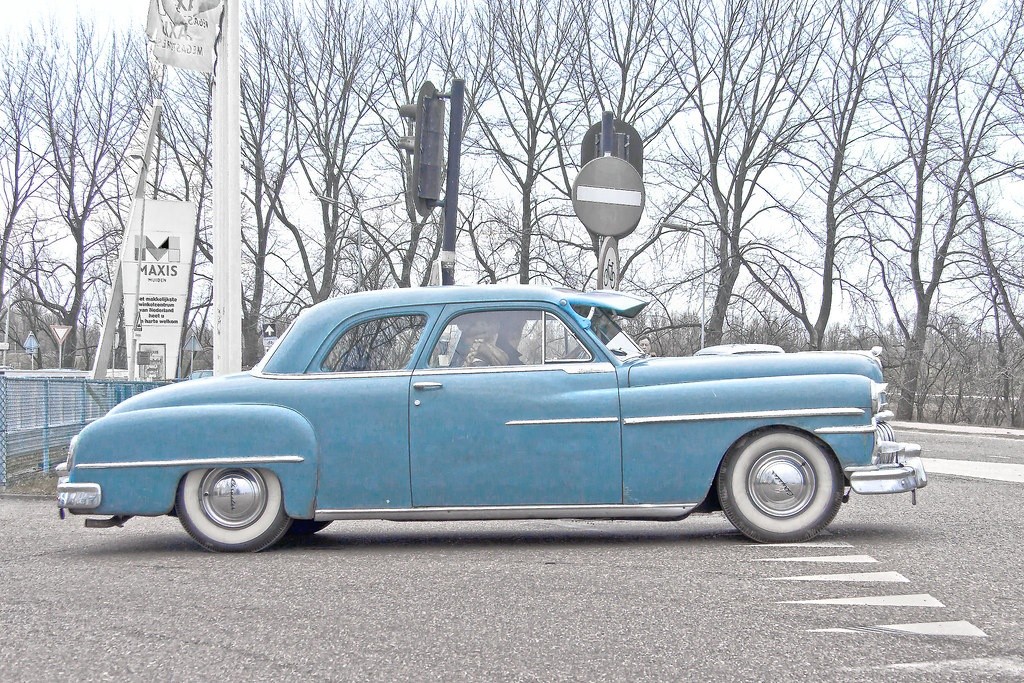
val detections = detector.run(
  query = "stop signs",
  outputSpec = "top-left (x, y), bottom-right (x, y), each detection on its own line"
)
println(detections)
top-left (572, 157), bottom-right (646, 238)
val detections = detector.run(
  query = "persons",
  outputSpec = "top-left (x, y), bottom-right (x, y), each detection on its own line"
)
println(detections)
top-left (456, 311), bottom-right (510, 369)
top-left (637, 336), bottom-right (657, 357)
top-left (493, 315), bottom-right (529, 365)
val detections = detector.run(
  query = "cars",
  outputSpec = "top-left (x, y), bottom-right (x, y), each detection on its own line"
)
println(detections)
top-left (692, 343), bottom-right (785, 356)
top-left (54, 285), bottom-right (927, 554)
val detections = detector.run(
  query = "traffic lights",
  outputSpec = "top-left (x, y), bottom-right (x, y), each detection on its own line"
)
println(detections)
top-left (397, 81), bottom-right (445, 217)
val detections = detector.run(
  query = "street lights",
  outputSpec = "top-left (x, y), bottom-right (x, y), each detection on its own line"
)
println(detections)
top-left (660, 222), bottom-right (706, 349)
top-left (319, 196), bottom-right (403, 292)
